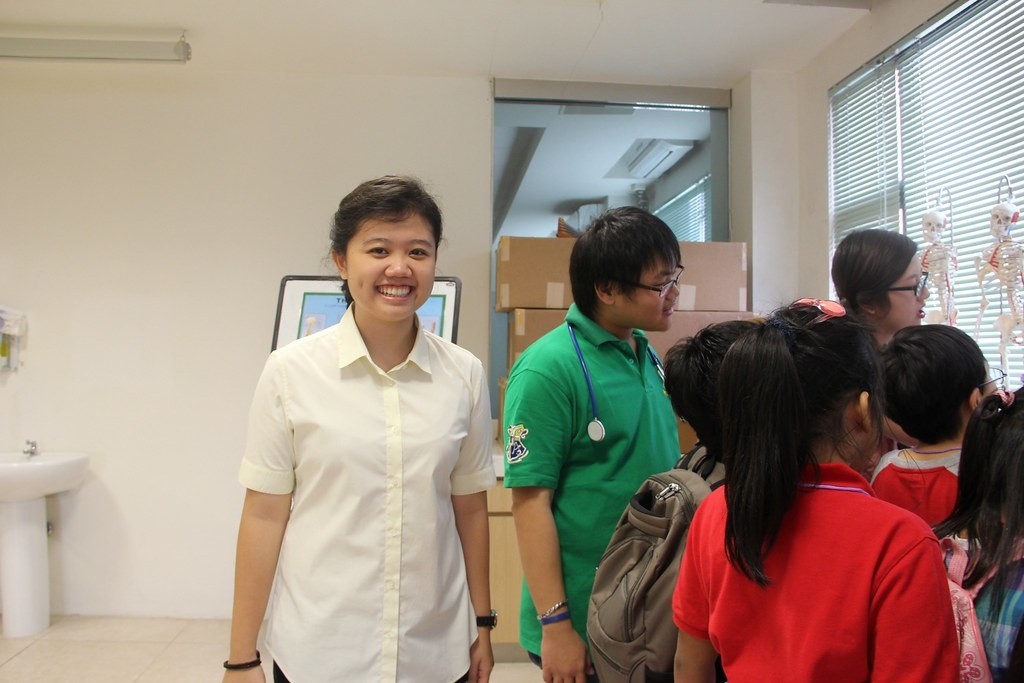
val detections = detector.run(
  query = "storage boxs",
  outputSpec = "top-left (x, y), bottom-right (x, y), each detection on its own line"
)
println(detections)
top-left (638, 310), bottom-right (756, 456)
top-left (507, 308), bottom-right (568, 380)
top-left (494, 234), bottom-right (578, 311)
top-left (671, 236), bottom-right (748, 311)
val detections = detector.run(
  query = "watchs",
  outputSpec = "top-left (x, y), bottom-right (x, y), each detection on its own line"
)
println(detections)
top-left (477, 609), bottom-right (498, 630)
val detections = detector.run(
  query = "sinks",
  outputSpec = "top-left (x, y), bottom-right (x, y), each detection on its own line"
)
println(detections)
top-left (0, 452), bottom-right (85, 503)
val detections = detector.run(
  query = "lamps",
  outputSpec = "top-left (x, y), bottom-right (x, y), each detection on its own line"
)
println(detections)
top-left (0, 26), bottom-right (192, 64)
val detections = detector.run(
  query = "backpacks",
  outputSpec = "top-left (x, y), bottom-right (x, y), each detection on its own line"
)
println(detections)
top-left (937, 505), bottom-right (1023, 683)
top-left (585, 445), bottom-right (728, 683)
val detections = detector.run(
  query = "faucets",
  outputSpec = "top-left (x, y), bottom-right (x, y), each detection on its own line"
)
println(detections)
top-left (22, 438), bottom-right (37, 455)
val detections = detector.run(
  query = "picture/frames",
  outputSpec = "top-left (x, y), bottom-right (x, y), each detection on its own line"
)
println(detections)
top-left (270, 274), bottom-right (461, 349)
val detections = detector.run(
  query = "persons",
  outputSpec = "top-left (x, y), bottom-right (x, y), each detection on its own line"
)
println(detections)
top-left (504, 205), bottom-right (685, 683)
top-left (220, 175), bottom-right (498, 683)
top-left (663, 229), bottom-right (1024, 683)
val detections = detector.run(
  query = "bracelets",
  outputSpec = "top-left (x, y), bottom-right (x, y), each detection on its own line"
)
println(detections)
top-left (540, 609), bottom-right (570, 625)
top-left (224, 649), bottom-right (261, 670)
top-left (536, 598), bottom-right (568, 621)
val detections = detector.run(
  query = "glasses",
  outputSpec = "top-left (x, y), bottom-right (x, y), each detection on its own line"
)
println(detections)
top-left (979, 368), bottom-right (1008, 387)
top-left (889, 271), bottom-right (932, 296)
top-left (632, 264), bottom-right (686, 298)
